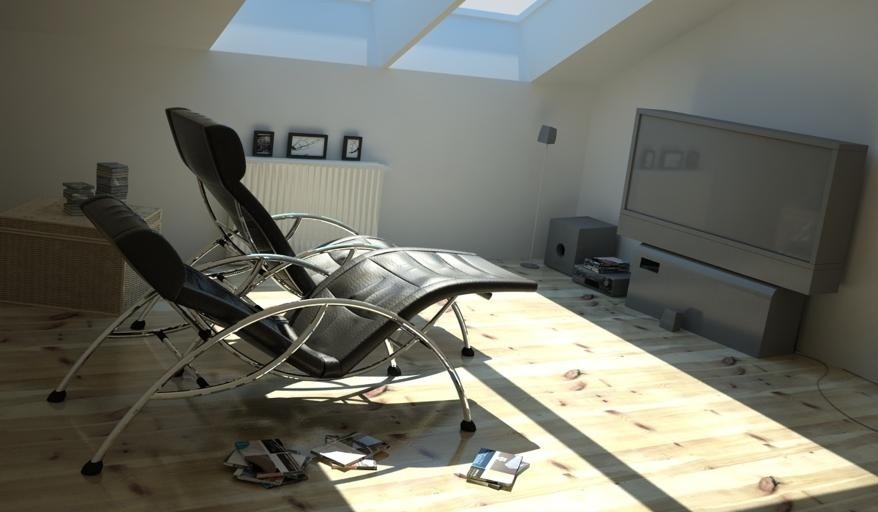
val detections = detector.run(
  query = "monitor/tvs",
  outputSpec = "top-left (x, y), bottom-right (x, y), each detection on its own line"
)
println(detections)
top-left (616, 107), bottom-right (869, 296)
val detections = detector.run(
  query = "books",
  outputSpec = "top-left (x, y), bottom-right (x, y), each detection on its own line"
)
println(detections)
top-left (584, 257), bottom-right (630, 275)
top-left (221, 438), bottom-right (311, 490)
top-left (63, 163), bottom-right (128, 217)
top-left (454, 447), bottom-right (530, 490)
top-left (311, 431), bottom-right (391, 470)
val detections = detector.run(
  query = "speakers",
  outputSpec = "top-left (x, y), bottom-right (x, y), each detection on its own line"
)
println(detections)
top-left (544, 213), bottom-right (615, 276)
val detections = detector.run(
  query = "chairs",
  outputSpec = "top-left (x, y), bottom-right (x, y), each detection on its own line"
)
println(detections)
top-left (129, 107), bottom-right (474, 377)
top-left (47, 194), bottom-right (539, 478)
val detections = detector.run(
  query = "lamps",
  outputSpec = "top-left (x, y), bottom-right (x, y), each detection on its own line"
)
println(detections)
top-left (521, 123), bottom-right (558, 270)
